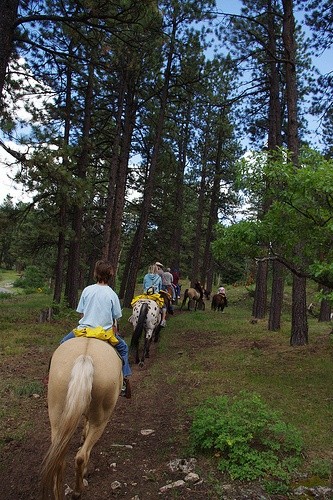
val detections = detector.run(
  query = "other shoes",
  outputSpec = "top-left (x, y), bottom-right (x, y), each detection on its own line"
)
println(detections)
top-left (160, 321), bottom-right (167, 328)
top-left (177, 295), bottom-right (181, 299)
top-left (172, 299), bottom-right (175, 301)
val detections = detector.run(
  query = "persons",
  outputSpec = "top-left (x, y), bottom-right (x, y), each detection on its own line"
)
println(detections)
top-left (47, 259), bottom-right (131, 398)
top-left (143, 264), bottom-right (166, 328)
top-left (193, 281), bottom-right (227, 308)
top-left (171, 267), bottom-right (180, 299)
top-left (163, 268), bottom-right (175, 302)
top-left (155, 262), bottom-right (169, 308)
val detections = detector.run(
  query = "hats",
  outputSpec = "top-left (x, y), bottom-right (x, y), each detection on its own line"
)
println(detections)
top-left (155, 262), bottom-right (163, 267)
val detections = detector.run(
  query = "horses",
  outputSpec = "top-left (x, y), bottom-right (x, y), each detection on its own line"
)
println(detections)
top-left (211, 294), bottom-right (229, 312)
top-left (128, 290), bottom-right (169, 366)
top-left (165, 271), bottom-right (179, 314)
top-left (179, 288), bottom-right (205, 311)
top-left (39, 337), bottom-right (124, 500)
